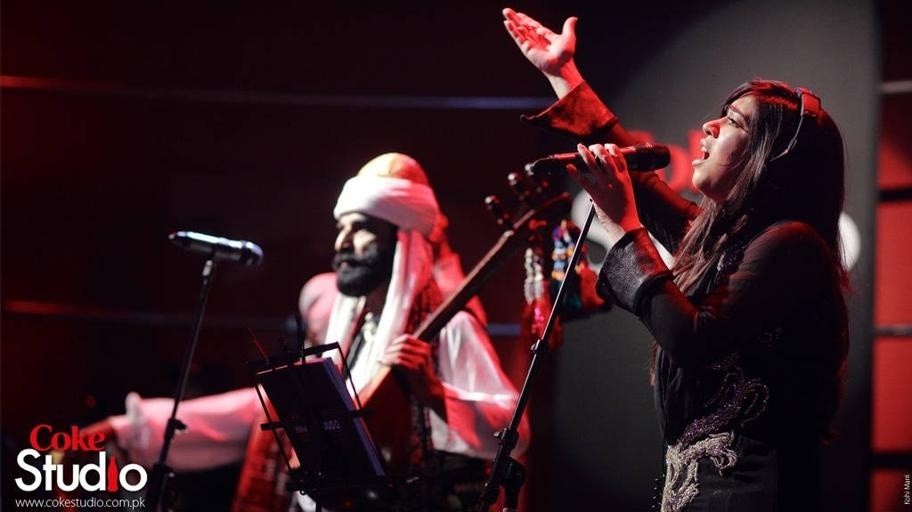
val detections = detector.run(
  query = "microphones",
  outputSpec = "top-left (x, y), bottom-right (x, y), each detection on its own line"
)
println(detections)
top-left (535, 145), bottom-right (672, 173)
top-left (168, 231), bottom-right (263, 267)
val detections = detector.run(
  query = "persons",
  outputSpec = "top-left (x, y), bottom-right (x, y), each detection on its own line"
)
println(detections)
top-left (50, 152), bottom-right (528, 512)
top-left (503, 8), bottom-right (851, 512)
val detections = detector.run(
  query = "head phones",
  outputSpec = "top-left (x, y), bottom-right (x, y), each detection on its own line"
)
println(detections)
top-left (754, 88), bottom-right (823, 199)
